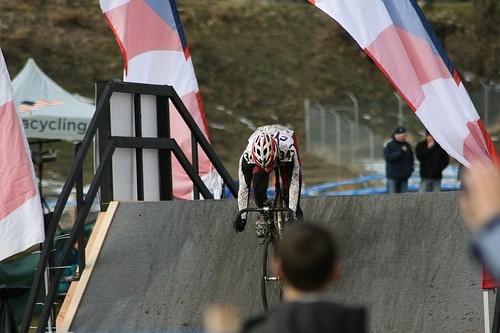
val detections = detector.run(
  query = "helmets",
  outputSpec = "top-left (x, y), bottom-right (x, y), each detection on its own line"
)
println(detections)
top-left (251, 131), bottom-right (277, 168)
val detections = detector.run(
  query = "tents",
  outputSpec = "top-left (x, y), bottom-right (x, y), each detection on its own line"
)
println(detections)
top-left (11, 58), bottom-right (102, 199)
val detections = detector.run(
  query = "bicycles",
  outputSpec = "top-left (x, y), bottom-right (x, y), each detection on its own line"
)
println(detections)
top-left (234, 165), bottom-right (295, 315)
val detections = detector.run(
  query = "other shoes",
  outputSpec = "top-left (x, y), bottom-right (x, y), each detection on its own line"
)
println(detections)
top-left (255, 211), bottom-right (267, 238)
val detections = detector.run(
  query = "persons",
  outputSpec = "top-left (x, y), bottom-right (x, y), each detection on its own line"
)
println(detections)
top-left (415, 129), bottom-right (449, 192)
top-left (234, 124), bottom-right (304, 235)
top-left (459, 156), bottom-right (500, 333)
top-left (204, 221), bottom-right (370, 333)
top-left (383, 127), bottom-right (414, 192)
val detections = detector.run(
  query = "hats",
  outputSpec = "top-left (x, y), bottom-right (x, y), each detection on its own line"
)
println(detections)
top-left (395, 127), bottom-right (407, 134)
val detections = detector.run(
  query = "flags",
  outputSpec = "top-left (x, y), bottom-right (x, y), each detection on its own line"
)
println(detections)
top-left (0, 48), bottom-right (46, 263)
top-left (99, 0), bottom-right (224, 201)
top-left (310, 0), bottom-right (500, 167)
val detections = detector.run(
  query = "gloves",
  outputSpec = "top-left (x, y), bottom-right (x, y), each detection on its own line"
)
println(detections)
top-left (232, 218), bottom-right (246, 232)
